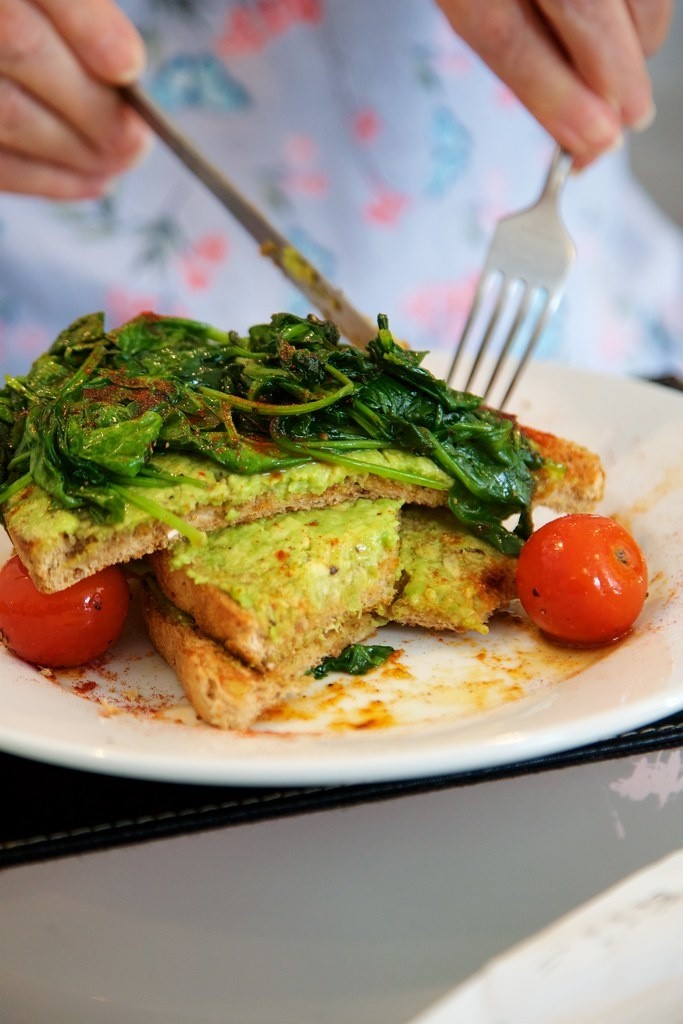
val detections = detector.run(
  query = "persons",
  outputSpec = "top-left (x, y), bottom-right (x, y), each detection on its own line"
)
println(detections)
top-left (1, 0), bottom-right (683, 391)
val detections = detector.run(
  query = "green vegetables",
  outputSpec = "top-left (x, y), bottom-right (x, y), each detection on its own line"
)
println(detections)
top-left (0, 312), bottom-right (545, 679)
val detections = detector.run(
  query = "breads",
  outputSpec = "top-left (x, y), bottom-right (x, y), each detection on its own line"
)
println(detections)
top-left (4, 423), bottom-right (606, 730)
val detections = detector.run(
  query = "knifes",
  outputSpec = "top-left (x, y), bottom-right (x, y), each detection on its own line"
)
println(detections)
top-left (121, 78), bottom-right (404, 355)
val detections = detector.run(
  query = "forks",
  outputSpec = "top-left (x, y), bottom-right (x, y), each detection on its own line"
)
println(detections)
top-left (445, 141), bottom-right (580, 410)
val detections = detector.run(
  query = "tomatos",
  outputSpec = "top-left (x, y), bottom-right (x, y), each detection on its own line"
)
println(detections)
top-left (517, 514), bottom-right (647, 644)
top-left (0, 552), bottom-right (130, 669)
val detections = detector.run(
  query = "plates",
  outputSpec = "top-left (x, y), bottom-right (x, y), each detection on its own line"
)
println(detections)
top-left (0, 362), bottom-right (683, 785)
